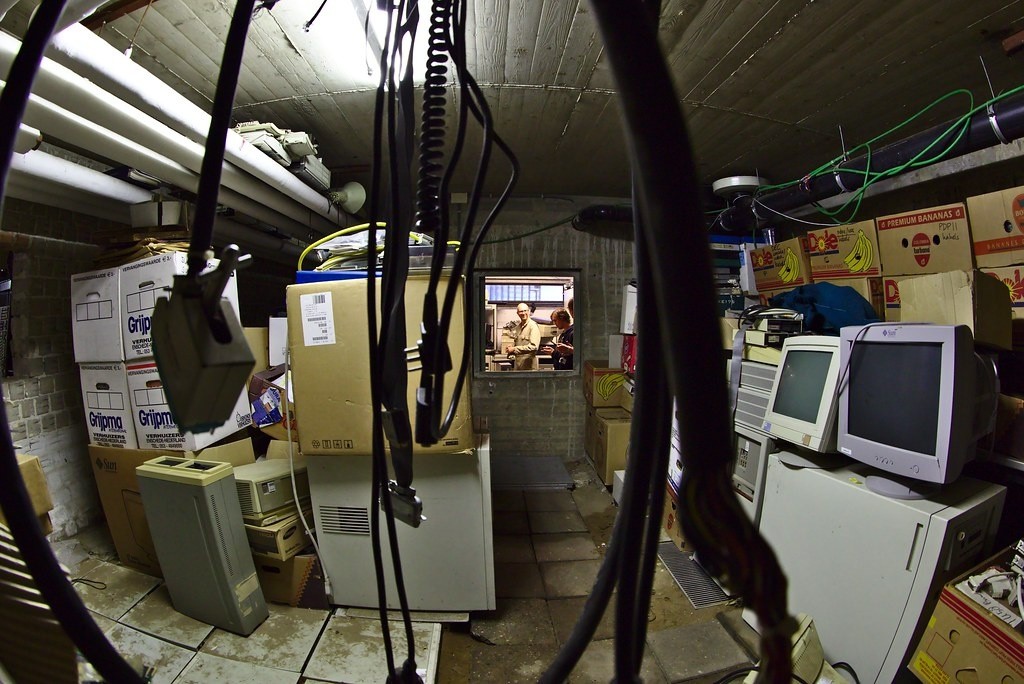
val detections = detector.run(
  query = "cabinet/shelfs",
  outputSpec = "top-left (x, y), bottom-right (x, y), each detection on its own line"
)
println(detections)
top-left (739, 137), bottom-right (1024, 471)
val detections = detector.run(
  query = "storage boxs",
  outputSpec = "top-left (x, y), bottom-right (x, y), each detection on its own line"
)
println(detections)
top-left (584, 284), bottom-right (638, 506)
top-left (70, 251), bottom-right (330, 609)
top-left (662, 395), bottom-right (695, 554)
top-left (720, 316), bottom-right (756, 350)
top-left (501, 336), bottom-right (516, 354)
top-left (286, 274), bottom-right (478, 456)
top-left (0, 453), bottom-right (54, 537)
top-left (538, 324), bottom-right (559, 337)
top-left (737, 186), bottom-right (1024, 462)
top-left (408, 244), bottom-right (457, 269)
top-left (907, 539), bottom-right (1024, 684)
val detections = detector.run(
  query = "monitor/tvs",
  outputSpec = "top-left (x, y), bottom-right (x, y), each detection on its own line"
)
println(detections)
top-left (837, 324), bottom-right (1000, 501)
top-left (762, 335), bottom-right (842, 469)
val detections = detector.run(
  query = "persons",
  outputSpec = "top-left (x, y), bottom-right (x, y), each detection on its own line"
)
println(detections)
top-left (542, 307), bottom-right (569, 370)
top-left (554, 299), bottom-right (574, 370)
top-left (506, 302), bottom-right (540, 370)
